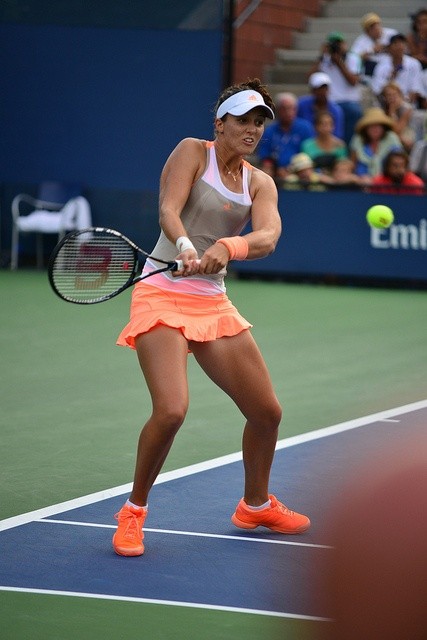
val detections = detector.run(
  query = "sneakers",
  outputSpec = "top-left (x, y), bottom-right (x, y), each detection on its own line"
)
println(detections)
top-left (112, 502), bottom-right (148, 556)
top-left (231, 494), bottom-right (311, 534)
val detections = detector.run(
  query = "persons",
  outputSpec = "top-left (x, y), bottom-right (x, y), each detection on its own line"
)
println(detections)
top-left (254, 8), bottom-right (427, 195)
top-left (111, 78), bottom-right (311, 557)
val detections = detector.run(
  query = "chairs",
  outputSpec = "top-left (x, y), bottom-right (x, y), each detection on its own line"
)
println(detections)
top-left (10, 194), bottom-right (78, 272)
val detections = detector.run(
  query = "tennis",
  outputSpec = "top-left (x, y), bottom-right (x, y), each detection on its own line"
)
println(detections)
top-left (366, 203), bottom-right (393, 232)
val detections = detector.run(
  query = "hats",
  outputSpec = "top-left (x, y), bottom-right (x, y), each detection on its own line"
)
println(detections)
top-left (356, 109), bottom-right (397, 131)
top-left (363, 13), bottom-right (381, 30)
top-left (217, 89), bottom-right (275, 120)
top-left (309, 72), bottom-right (332, 90)
top-left (386, 33), bottom-right (406, 43)
top-left (291, 153), bottom-right (314, 172)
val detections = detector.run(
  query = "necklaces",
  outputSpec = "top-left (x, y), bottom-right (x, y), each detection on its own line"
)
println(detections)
top-left (216, 153), bottom-right (243, 182)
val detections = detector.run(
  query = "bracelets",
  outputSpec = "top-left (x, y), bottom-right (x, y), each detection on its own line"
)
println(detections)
top-left (216, 235), bottom-right (248, 261)
top-left (175, 236), bottom-right (198, 253)
